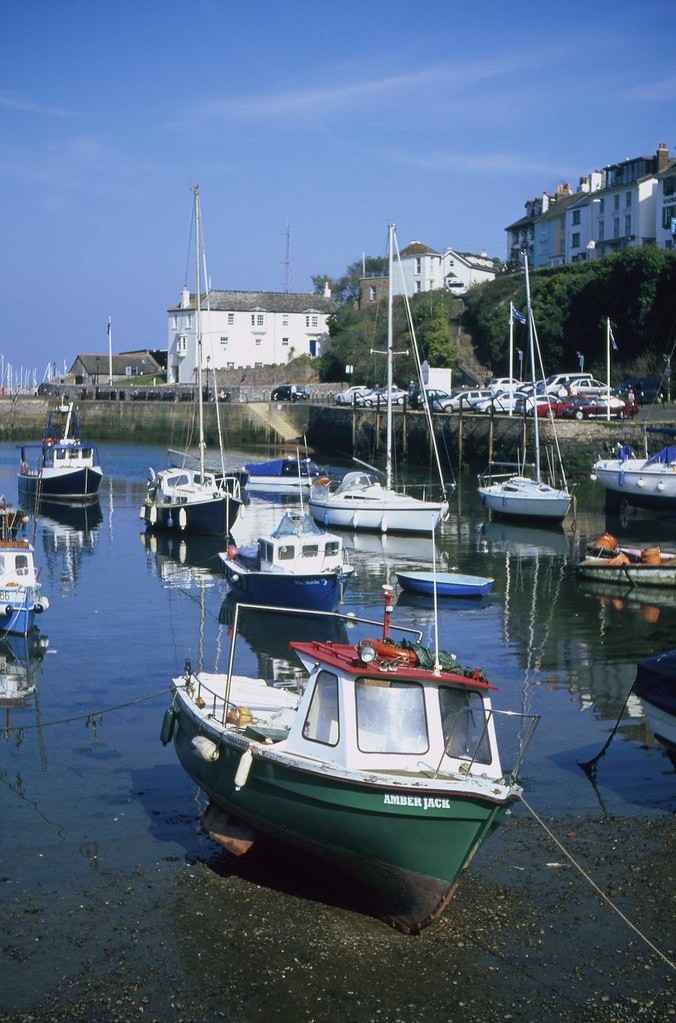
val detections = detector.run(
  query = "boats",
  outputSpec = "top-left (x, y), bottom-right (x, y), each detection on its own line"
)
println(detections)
top-left (576, 532), bottom-right (676, 586)
top-left (395, 570), bottom-right (495, 597)
top-left (1, 448), bottom-right (50, 634)
top-left (0, 627), bottom-right (50, 769)
top-left (636, 650), bottom-right (676, 753)
top-left (160, 516), bottom-right (542, 934)
top-left (18, 390), bottom-right (104, 500)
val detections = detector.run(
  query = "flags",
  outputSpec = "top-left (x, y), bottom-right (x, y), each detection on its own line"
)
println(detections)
top-left (512, 306), bottom-right (526, 324)
top-left (610, 329), bottom-right (618, 349)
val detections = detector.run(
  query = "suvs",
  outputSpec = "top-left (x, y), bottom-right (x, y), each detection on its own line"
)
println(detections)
top-left (487, 377), bottom-right (525, 394)
top-left (612, 377), bottom-right (667, 404)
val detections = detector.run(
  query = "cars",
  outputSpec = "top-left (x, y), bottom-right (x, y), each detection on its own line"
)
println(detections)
top-left (433, 379), bottom-right (639, 419)
top-left (335, 386), bottom-right (448, 410)
top-left (271, 385), bottom-right (307, 403)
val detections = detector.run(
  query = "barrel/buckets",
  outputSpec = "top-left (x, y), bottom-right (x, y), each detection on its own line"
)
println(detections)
top-left (595, 532), bottom-right (617, 550)
top-left (641, 547), bottom-right (661, 564)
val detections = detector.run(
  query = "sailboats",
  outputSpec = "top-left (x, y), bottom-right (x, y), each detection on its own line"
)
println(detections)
top-left (590, 334), bottom-right (676, 497)
top-left (476, 249), bottom-right (581, 517)
top-left (141, 186), bottom-right (451, 611)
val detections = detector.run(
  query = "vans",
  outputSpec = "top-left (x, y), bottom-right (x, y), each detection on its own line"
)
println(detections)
top-left (530, 373), bottom-right (593, 396)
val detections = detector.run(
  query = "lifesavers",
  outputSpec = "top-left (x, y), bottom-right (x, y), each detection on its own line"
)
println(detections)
top-left (364, 639), bottom-right (419, 663)
top-left (318, 478), bottom-right (329, 487)
top-left (46, 438), bottom-right (54, 446)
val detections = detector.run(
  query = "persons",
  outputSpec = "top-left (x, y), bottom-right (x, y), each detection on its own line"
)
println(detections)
top-left (628, 390), bottom-right (635, 403)
top-left (290, 384), bottom-right (297, 403)
